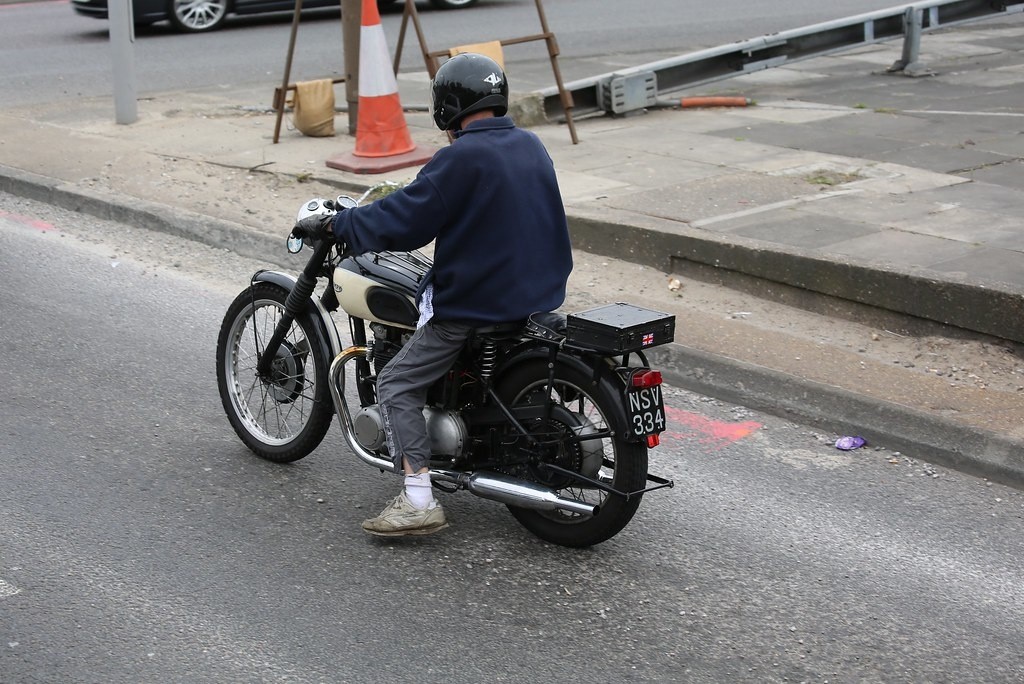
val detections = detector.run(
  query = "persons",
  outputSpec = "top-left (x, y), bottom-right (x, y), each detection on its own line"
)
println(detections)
top-left (292, 53), bottom-right (573, 536)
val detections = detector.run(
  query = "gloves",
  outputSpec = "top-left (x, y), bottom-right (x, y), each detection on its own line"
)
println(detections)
top-left (291, 214), bottom-right (335, 252)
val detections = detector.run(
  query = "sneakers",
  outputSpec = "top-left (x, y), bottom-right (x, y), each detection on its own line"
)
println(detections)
top-left (360, 488), bottom-right (448, 538)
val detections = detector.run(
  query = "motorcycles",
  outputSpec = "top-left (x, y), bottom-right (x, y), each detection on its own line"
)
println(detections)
top-left (215, 181), bottom-right (676, 549)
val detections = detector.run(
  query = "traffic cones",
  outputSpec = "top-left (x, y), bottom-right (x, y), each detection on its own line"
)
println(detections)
top-left (325, 0), bottom-right (438, 175)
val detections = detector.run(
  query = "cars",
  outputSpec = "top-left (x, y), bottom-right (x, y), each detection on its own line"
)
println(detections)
top-left (70, 0), bottom-right (489, 34)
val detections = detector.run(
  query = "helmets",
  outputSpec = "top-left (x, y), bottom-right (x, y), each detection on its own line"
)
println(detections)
top-left (428, 52), bottom-right (509, 131)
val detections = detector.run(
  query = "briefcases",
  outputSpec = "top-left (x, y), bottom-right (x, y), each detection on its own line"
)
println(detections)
top-left (566, 300), bottom-right (676, 355)
